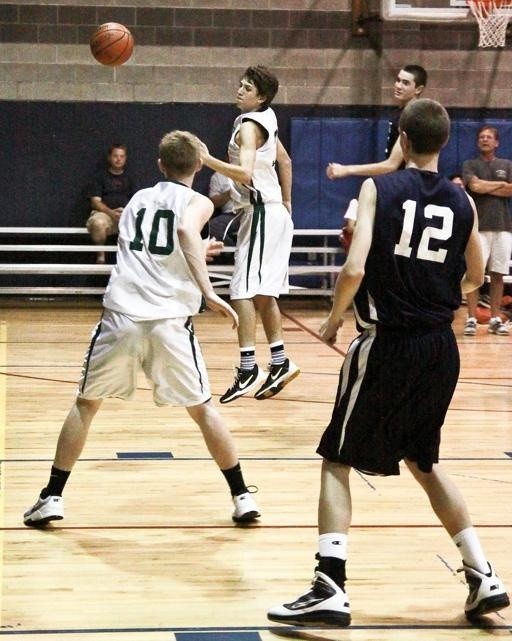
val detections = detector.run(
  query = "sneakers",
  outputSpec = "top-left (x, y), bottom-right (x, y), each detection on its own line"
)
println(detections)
top-left (266, 567), bottom-right (352, 626)
top-left (486, 317), bottom-right (511, 336)
top-left (255, 357), bottom-right (301, 400)
top-left (230, 491), bottom-right (261, 522)
top-left (22, 487), bottom-right (65, 525)
top-left (219, 362), bottom-right (266, 405)
top-left (462, 557), bottom-right (511, 618)
top-left (463, 316), bottom-right (479, 336)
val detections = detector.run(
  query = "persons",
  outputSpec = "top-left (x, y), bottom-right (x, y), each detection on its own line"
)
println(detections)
top-left (326, 65), bottom-right (428, 181)
top-left (267, 96), bottom-right (511, 623)
top-left (24, 133), bottom-right (260, 523)
top-left (195, 64), bottom-right (302, 402)
top-left (205, 147), bottom-right (244, 241)
top-left (450, 174), bottom-right (465, 191)
top-left (340, 194), bottom-right (372, 332)
top-left (461, 124), bottom-right (512, 335)
top-left (88, 142), bottom-right (140, 264)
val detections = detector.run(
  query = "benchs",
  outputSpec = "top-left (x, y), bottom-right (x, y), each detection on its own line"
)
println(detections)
top-left (485, 260), bottom-right (512, 284)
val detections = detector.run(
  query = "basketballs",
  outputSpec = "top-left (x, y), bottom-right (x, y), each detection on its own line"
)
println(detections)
top-left (90, 23), bottom-right (134, 66)
